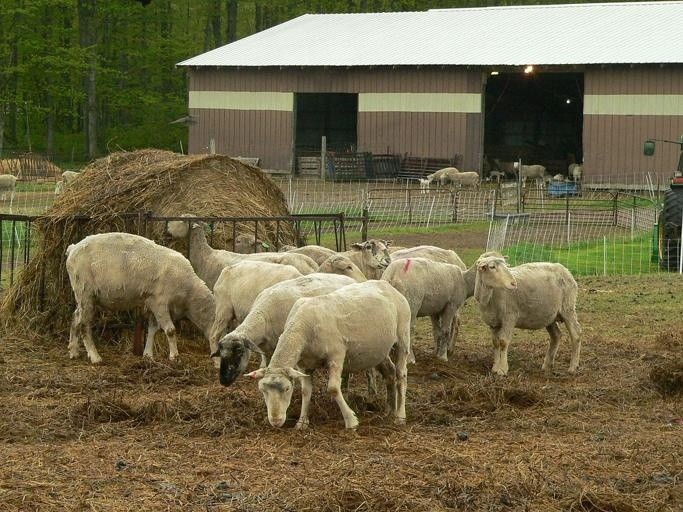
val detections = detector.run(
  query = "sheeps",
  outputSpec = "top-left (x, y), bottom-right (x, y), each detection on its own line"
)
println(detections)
top-left (417, 166), bottom-right (480, 194)
top-left (211, 271), bottom-right (381, 399)
top-left (242, 280), bottom-right (412, 436)
top-left (0, 173), bottom-right (16, 201)
top-left (64, 231), bottom-right (239, 368)
top-left (53, 181), bottom-right (62, 195)
top-left (488, 158), bottom-right (582, 188)
top-left (473, 256), bottom-right (584, 376)
top-left (166, 213), bottom-right (510, 366)
top-left (61, 171), bottom-right (80, 187)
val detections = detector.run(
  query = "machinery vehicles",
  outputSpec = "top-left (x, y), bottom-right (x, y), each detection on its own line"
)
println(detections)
top-left (644, 133), bottom-right (683, 271)
